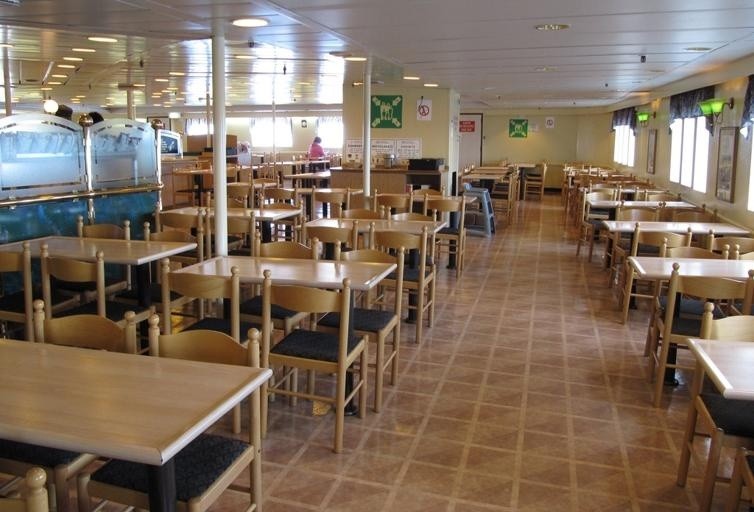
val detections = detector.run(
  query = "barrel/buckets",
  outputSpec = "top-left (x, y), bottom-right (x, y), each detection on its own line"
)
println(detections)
top-left (382, 151), bottom-right (397, 168)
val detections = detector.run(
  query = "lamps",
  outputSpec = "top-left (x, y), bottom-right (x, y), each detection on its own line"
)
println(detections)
top-left (44, 100), bottom-right (58, 125)
top-left (697, 97), bottom-right (734, 124)
top-left (635, 111), bottom-right (656, 127)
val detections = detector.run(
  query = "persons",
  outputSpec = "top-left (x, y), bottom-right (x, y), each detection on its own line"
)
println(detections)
top-left (309, 136), bottom-right (325, 161)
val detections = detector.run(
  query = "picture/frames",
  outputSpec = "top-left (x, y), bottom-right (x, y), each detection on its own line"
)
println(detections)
top-left (715, 127), bottom-right (739, 203)
top-left (647, 129), bottom-right (657, 174)
